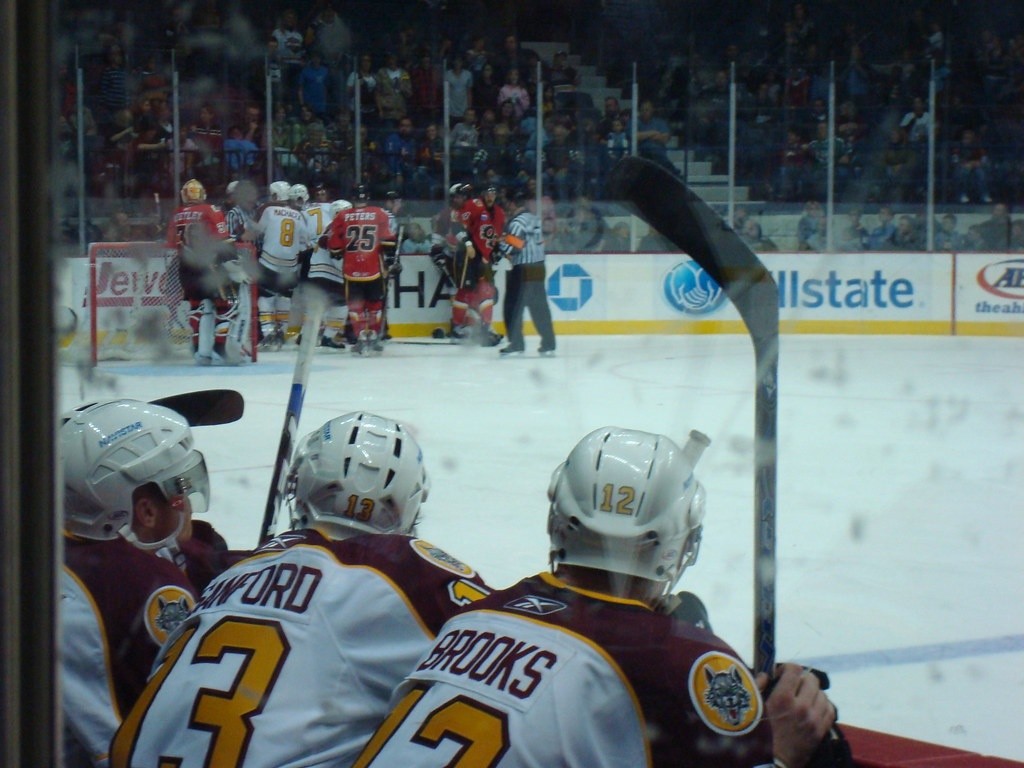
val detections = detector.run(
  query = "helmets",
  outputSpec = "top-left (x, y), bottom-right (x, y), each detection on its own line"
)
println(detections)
top-left (385, 191), bottom-right (400, 201)
top-left (449, 182), bottom-right (473, 198)
top-left (181, 179), bottom-right (208, 206)
top-left (290, 184), bottom-right (310, 202)
top-left (225, 181), bottom-right (239, 195)
top-left (353, 185), bottom-right (370, 203)
top-left (270, 181), bottom-right (291, 202)
top-left (58, 398), bottom-right (211, 554)
top-left (479, 181), bottom-right (498, 196)
top-left (545, 425), bottom-right (712, 598)
top-left (283, 410), bottom-right (430, 542)
top-left (328, 199), bottom-right (353, 218)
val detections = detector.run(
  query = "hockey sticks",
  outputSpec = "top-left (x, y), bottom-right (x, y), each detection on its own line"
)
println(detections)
top-left (601, 153), bottom-right (782, 677)
top-left (252, 294), bottom-right (334, 546)
top-left (146, 387), bottom-right (244, 427)
top-left (376, 225), bottom-right (406, 341)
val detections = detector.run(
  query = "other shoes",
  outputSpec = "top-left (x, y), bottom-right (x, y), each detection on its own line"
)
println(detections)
top-left (321, 337), bottom-right (345, 348)
top-left (296, 334), bottom-right (302, 344)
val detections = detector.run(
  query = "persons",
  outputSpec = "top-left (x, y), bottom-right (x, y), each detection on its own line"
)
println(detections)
top-left (48, 0), bottom-right (1024, 362)
top-left (54, 398), bottom-right (837, 768)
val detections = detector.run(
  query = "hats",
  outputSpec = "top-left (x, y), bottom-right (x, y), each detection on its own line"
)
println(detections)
top-left (508, 188), bottom-right (527, 201)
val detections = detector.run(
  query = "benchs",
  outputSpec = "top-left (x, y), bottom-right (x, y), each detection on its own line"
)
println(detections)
top-left (43, 2), bottom-right (1023, 255)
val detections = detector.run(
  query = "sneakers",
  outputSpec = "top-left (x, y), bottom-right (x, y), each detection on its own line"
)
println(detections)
top-left (446, 319), bottom-right (473, 343)
top-left (500, 341), bottom-right (525, 357)
top-left (537, 345), bottom-right (556, 357)
top-left (367, 330), bottom-right (385, 356)
top-left (479, 331), bottom-right (504, 347)
top-left (351, 330), bottom-right (368, 357)
